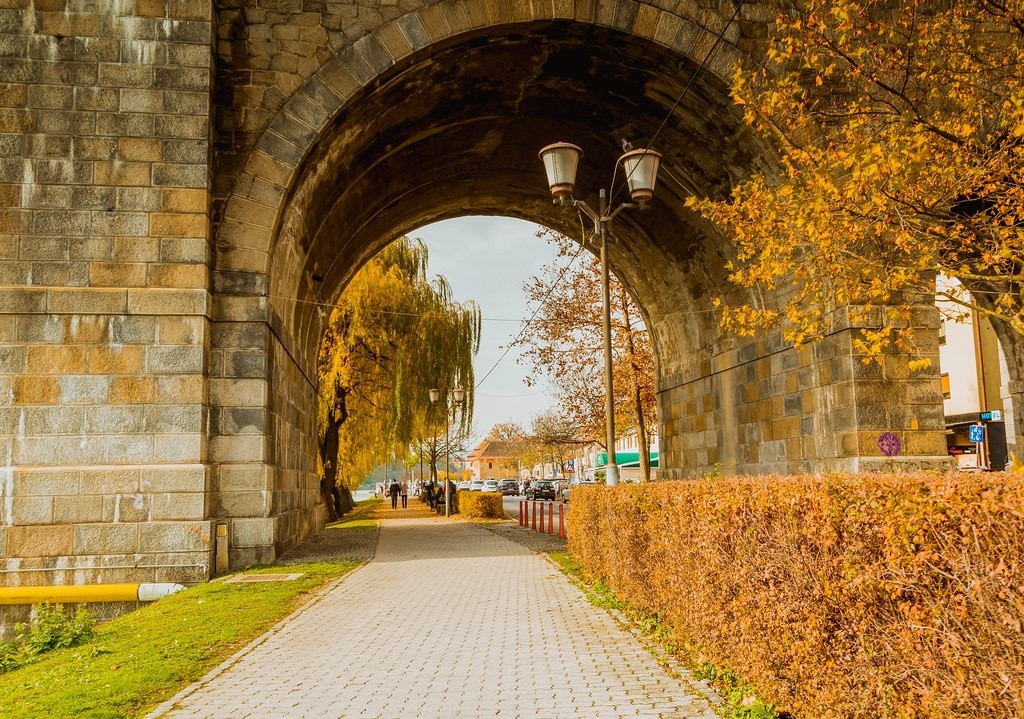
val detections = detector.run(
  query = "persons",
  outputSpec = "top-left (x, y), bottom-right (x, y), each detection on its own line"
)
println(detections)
top-left (389, 479), bottom-right (400, 509)
top-left (435, 487), bottom-right (446, 509)
top-left (380, 480), bottom-right (428, 499)
top-left (444, 477), bottom-right (454, 514)
top-left (400, 480), bottom-right (409, 508)
top-left (523, 477), bottom-right (538, 497)
top-left (423, 480), bottom-right (435, 512)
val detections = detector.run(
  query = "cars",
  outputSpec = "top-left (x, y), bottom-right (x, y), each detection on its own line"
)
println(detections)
top-left (487, 477), bottom-right (495, 480)
top-left (468, 480), bottom-right (482, 492)
top-left (519, 480), bottom-right (529, 496)
top-left (561, 487), bottom-right (571, 503)
top-left (480, 480), bottom-right (499, 492)
top-left (436, 480), bottom-right (470, 491)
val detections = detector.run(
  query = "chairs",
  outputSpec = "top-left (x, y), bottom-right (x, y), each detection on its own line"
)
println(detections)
top-left (437, 494), bottom-right (446, 515)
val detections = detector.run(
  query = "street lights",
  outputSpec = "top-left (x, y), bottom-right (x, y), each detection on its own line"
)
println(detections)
top-left (535, 136), bottom-right (664, 484)
top-left (428, 386), bottom-right (466, 517)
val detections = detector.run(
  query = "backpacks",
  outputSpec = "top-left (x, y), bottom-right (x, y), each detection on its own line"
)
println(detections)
top-left (449, 480), bottom-right (456, 494)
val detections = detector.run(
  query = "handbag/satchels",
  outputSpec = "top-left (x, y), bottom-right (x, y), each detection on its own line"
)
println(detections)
top-left (431, 490), bottom-right (437, 497)
top-left (439, 494), bottom-right (443, 501)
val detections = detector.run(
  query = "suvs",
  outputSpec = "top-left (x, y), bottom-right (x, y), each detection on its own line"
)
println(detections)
top-left (525, 481), bottom-right (555, 501)
top-left (498, 478), bottom-right (519, 497)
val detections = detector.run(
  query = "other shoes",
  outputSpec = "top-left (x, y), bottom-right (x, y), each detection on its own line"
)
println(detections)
top-left (450, 511), bottom-right (453, 515)
top-left (430, 508), bottom-right (432, 511)
top-left (432, 510), bottom-right (436, 512)
